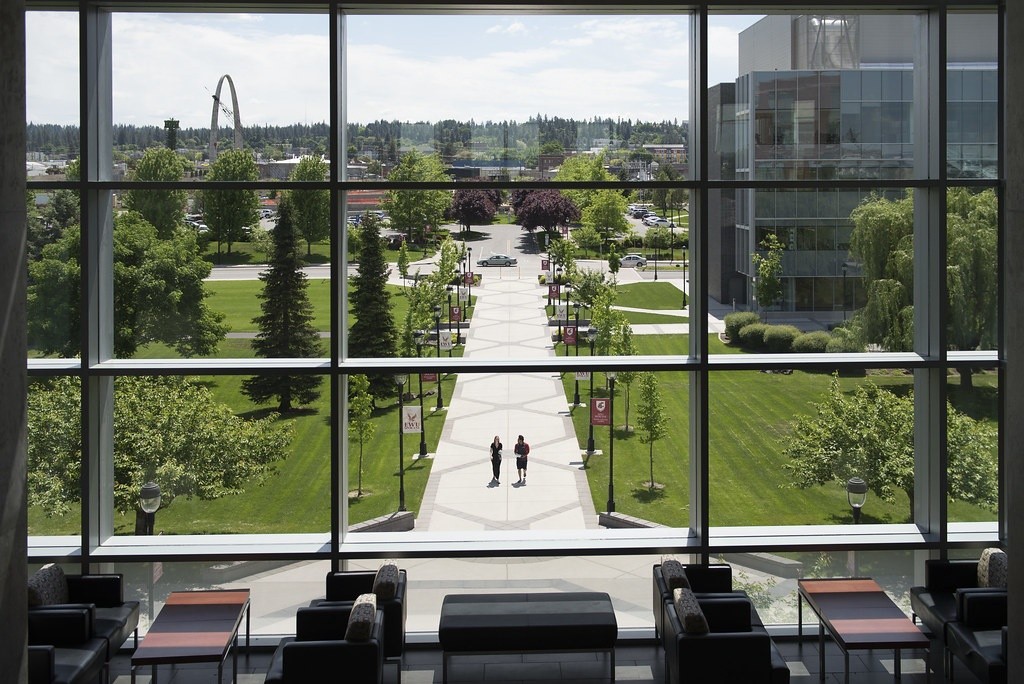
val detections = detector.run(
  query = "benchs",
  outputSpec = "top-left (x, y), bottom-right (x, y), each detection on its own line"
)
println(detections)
top-left (437, 591), bottom-right (618, 684)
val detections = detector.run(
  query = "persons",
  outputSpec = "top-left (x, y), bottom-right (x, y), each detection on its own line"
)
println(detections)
top-left (514, 435), bottom-right (529, 485)
top-left (490, 436), bottom-right (503, 484)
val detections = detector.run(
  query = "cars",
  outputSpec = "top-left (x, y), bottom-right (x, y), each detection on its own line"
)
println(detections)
top-left (347, 211), bottom-right (384, 227)
top-left (476, 254), bottom-right (517, 267)
top-left (184, 213), bottom-right (208, 234)
top-left (258, 208), bottom-right (274, 220)
top-left (628, 206), bottom-right (677, 228)
top-left (618, 255), bottom-right (647, 268)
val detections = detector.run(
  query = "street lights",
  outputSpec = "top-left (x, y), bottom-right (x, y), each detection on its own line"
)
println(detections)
top-left (433, 304), bottom-right (443, 408)
top-left (412, 333), bottom-right (427, 455)
top-left (461, 255), bottom-right (468, 323)
top-left (681, 245), bottom-right (687, 307)
top-left (453, 269), bottom-right (462, 345)
top-left (564, 282), bottom-right (571, 356)
top-left (466, 246), bottom-right (472, 307)
top-left (395, 373), bottom-right (407, 512)
top-left (845, 475), bottom-right (869, 580)
top-left (446, 286), bottom-right (453, 357)
top-left (571, 303), bottom-right (581, 405)
top-left (605, 371), bottom-right (617, 513)
top-left (587, 328), bottom-right (598, 452)
top-left (139, 482), bottom-right (163, 621)
top-left (653, 232), bottom-right (658, 280)
top-left (547, 245), bottom-right (552, 305)
top-left (551, 254), bottom-right (558, 317)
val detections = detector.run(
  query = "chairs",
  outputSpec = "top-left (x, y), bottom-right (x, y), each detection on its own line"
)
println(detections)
top-left (27, 547), bottom-right (1013, 684)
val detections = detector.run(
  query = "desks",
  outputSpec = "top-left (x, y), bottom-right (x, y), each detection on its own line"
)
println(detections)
top-left (796, 577), bottom-right (931, 684)
top-left (129, 588), bottom-right (252, 684)
